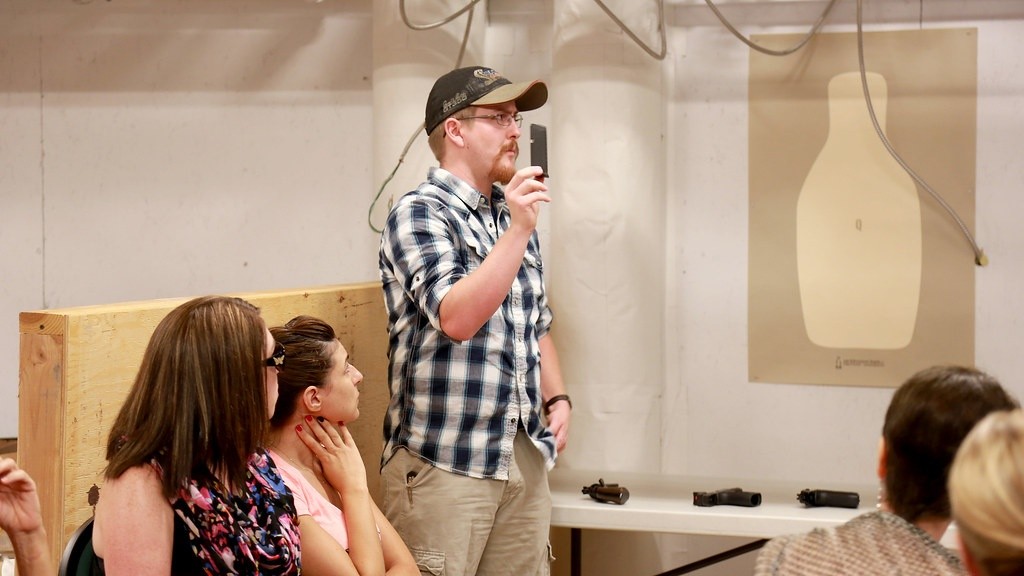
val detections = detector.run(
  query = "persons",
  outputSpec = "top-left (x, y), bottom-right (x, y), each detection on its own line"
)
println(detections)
top-left (376, 66), bottom-right (572, 576)
top-left (755, 367), bottom-right (1024, 576)
top-left (0, 294), bottom-right (427, 576)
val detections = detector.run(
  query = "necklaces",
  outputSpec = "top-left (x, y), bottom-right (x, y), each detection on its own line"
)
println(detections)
top-left (272, 442), bottom-right (330, 487)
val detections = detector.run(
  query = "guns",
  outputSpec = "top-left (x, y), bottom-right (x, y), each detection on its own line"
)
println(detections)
top-left (796, 488), bottom-right (861, 509)
top-left (582, 478), bottom-right (630, 506)
top-left (692, 488), bottom-right (762, 507)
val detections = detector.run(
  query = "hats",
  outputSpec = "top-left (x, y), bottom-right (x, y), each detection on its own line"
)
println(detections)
top-left (424, 65), bottom-right (550, 136)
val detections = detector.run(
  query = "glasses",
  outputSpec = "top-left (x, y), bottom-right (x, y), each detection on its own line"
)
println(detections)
top-left (261, 341), bottom-right (287, 369)
top-left (443, 111), bottom-right (524, 138)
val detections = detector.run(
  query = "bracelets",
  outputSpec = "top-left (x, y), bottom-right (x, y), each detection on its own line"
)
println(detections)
top-left (544, 394), bottom-right (571, 416)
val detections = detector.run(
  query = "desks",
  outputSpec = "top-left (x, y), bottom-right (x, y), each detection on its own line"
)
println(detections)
top-left (550, 491), bottom-right (962, 576)
